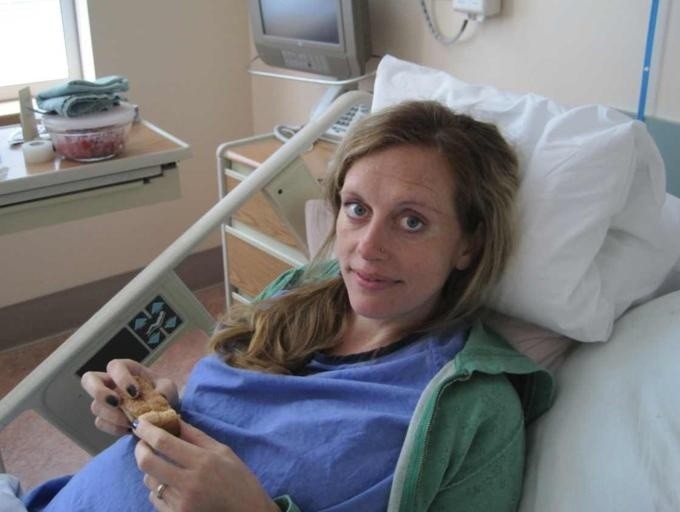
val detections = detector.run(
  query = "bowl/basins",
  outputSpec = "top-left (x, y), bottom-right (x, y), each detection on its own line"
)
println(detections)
top-left (41, 111), bottom-right (137, 162)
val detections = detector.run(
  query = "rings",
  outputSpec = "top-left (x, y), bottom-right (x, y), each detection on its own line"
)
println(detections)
top-left (155, 484), bottom-right (168, 501)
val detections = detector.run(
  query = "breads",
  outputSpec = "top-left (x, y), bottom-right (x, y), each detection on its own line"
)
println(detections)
top-left (112, 376), bottom-right (177, 435)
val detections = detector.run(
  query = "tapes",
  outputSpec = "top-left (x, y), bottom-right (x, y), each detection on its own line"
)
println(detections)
top-left (20, 139), bottom-right (55, 165)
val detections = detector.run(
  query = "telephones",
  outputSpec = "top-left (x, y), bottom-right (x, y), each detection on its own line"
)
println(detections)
top-left (308, 84), bottom-right (370, 141)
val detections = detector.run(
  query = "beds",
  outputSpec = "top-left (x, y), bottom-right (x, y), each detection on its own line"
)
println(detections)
top-left (2, 91), bottom-right (678, 512)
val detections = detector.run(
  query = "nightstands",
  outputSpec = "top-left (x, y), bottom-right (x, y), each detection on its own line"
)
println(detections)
top-left (216, 131), bottom-right (339, 318)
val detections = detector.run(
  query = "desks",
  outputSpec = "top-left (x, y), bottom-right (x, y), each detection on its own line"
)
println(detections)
top-left (1, 117), bottom-right (194, 235)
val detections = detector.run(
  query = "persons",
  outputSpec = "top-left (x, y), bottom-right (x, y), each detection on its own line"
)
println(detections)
top-left (19, 98), bottom-right (555, 512)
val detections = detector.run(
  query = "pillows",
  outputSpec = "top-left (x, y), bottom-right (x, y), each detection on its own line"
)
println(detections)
top-left (369, 54), bottom-right (679, 345)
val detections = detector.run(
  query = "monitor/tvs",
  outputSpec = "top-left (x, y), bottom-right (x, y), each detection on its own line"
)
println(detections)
top-left (249, 0), bottom-right (372, 79)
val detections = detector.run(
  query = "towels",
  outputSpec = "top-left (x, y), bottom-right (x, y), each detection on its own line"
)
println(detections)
top-left (35, 74), bottom-right (130, 117)
